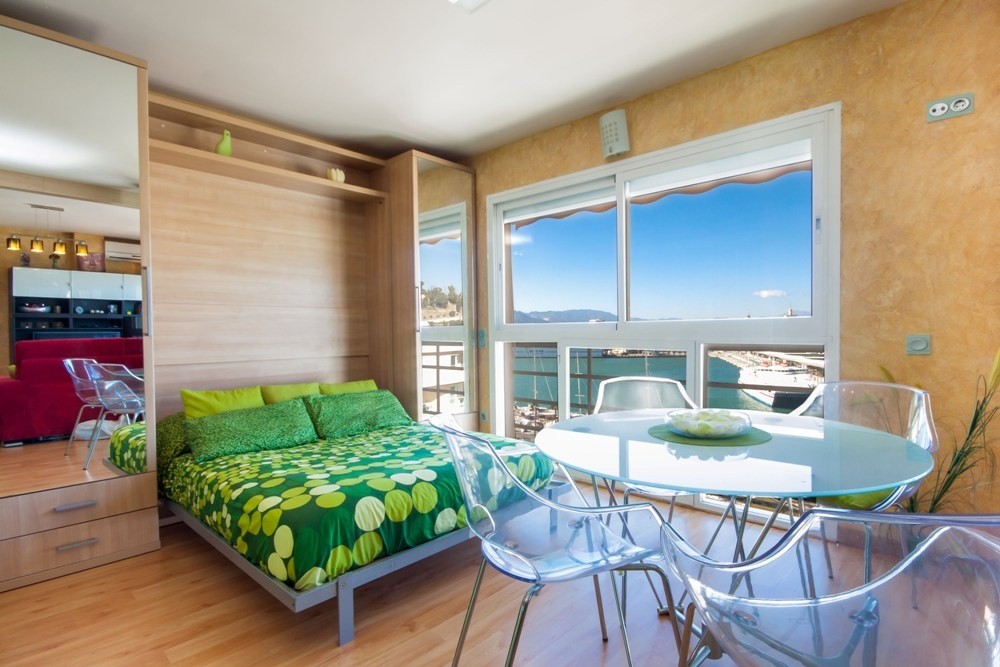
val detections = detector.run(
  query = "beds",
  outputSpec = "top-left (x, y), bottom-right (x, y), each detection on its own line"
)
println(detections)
top-left (156, 389), bottom-right (572, 647)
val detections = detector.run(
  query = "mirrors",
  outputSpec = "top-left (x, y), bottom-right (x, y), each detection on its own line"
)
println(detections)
top-left (0, 24), bottom-right (147, 498)
top-left (417, 157), bottom-right (477, 415)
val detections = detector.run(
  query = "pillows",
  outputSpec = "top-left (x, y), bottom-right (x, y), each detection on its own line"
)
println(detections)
top-left (182, 379), bottom-right (412, 463)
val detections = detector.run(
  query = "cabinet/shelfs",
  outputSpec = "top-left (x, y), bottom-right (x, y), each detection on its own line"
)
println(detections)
top-left (0, 469), bottom-right (160, 592)
top-left (149, 94), bottom-right (388, 199)
top-left (8, 266), bottom-right (142, 340)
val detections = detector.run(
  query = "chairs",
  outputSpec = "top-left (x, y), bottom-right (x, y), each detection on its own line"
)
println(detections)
top-left (423, 423), bottom-right (678, 667)
top-left (788, 381), bottom-right (938, 610)
top-left (62, 358), bottom-right (145, 470)
top-left (661, 508), bottom-right (1000, 667)
top-left (592, 375), bottom-right (700, 545)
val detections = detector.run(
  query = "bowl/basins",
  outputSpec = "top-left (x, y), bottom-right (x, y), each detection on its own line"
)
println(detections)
top-left (665, 408), bottom-right (751, 438)
top-left (21, 305), bottom-right (51, 313)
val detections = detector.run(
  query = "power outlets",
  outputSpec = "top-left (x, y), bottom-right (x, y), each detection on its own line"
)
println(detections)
top-left (926, 92), bottom-right (973, 123)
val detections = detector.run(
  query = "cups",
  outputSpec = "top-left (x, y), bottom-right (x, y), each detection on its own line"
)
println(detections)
top-left (54, 306), bottom-right (61, 314)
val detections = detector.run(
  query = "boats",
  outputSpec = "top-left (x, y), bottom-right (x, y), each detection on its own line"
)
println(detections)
top-left (737, 356), bottom-right (822, 408)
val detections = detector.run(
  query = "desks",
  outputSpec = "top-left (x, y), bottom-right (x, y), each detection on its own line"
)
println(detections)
top-left (536, 407), bottom-right (934, 667)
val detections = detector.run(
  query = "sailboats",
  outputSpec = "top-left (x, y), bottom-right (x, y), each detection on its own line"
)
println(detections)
top-left (515, 347), bottom-right (665, 433)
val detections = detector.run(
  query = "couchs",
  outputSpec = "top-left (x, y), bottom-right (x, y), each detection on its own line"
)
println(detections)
top-left (0, 338), bottom-right (144, 447)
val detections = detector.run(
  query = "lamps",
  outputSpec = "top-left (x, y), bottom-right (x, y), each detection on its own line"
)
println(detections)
top-left (5, 204), bottom-right (89, 257)
